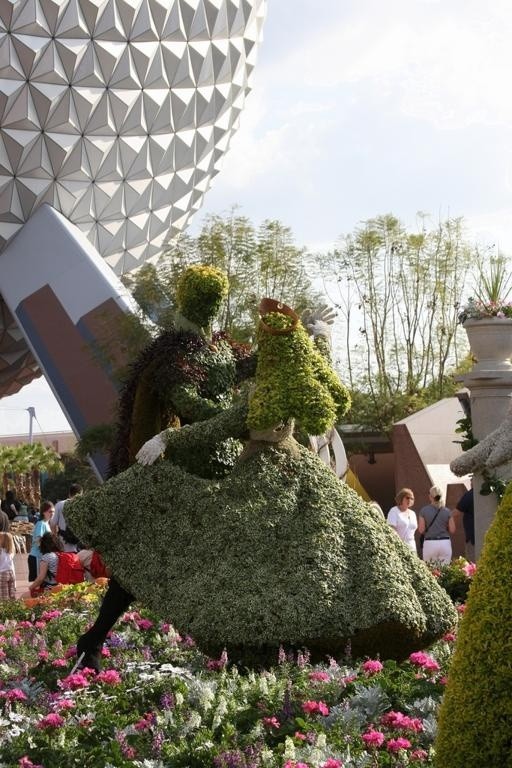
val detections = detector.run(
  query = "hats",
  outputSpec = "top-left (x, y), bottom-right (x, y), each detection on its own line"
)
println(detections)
top-left (429, 486), bottom-right (443, 499)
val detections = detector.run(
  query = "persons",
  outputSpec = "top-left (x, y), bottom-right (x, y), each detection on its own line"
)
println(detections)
top-left (0, 483), bottom-right (109, 600)
top-left (451, 480), bottom-right (474, 564)
top-left (418, 484), bottom-right (456, 565)
top-left (386, 488), bottom-right (418, 553)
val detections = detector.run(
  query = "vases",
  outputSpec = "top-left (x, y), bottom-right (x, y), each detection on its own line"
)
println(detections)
top-left (462, 317), bottom-right (511, 371)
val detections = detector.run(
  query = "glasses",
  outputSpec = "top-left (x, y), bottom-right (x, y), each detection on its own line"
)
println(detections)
top-left (403, 496), bottom-right (414, 500)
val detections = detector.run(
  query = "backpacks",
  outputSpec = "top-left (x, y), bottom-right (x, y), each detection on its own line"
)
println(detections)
top-left (57, 526), bottom-right (80, 545)
top-left (48, 551), bottom-right (85, 584)
top-left (84, 547), bottom-right (112, 578)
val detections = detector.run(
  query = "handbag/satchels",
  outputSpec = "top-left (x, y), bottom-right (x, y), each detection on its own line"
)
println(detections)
top-left (419, 533), bottom-right (425, 549)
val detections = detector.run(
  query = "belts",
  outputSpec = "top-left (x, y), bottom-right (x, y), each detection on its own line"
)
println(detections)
top-left (425, 536), bottom-right (449, 540)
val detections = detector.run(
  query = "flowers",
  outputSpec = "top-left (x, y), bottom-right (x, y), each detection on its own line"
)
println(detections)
top-left (451, 260), bottom-right (512, 322)
